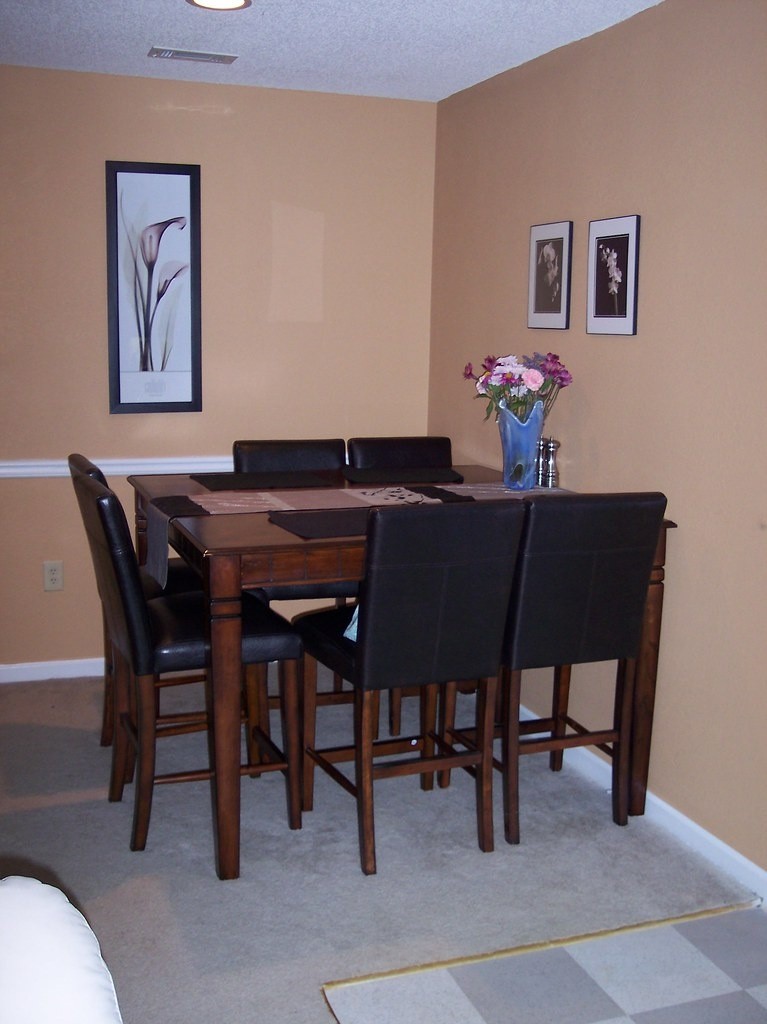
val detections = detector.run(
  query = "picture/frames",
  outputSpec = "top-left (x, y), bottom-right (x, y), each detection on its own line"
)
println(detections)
top-left (587, 214), bottom-right (638, 337)
top-left (105, 159), bottom-right (202, 414)
top-left (526, 221), bottom-right (572, 330)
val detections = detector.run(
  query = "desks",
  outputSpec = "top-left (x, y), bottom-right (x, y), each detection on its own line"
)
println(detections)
top-left (125, 465), bottom-right (678, 881)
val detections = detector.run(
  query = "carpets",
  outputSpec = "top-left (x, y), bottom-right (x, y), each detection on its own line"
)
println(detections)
top-left (318, 900), bottom-right (767, 1024)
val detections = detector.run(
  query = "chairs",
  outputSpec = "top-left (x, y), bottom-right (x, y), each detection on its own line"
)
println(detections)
top-left (234, 436), bottom-right (454, 737)
top-left (443, 490), bottom-right (670, 846)
top-left (292, 494), bottom-right (526, 875)
top-left (67, 454), bottom-right (307, 850)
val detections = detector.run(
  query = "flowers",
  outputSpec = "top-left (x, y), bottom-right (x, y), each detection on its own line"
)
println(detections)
top-left (464, 350), bottom-right (573, 424)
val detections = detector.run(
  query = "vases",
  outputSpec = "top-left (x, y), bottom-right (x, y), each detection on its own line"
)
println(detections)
top-left (494, 398), bottom-right (545, 491)
